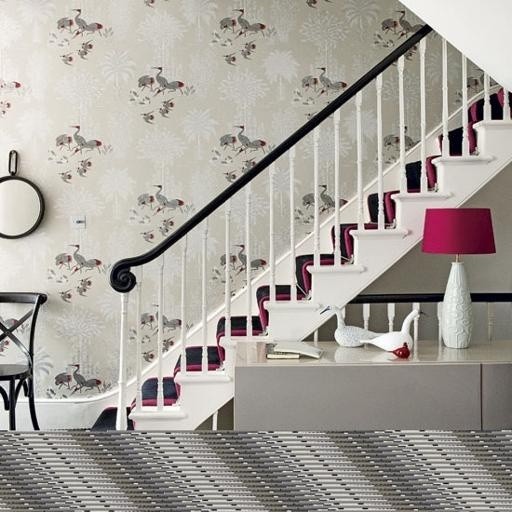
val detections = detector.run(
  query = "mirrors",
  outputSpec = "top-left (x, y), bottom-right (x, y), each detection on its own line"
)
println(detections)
top-left (0, 150), bottom-right (45, 240)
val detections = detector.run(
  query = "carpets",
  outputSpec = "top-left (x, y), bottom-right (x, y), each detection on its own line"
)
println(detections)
top-left (0, 426), bottom-right (512, 512)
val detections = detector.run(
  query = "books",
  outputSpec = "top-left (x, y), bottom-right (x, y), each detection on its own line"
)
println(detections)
top-left (273, 341), bottom-right (324, 360)
top-left (266, 353), bottom-right (300, 361)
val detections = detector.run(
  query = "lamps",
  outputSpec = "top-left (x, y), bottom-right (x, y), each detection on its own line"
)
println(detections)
top-left (419, 207), bottom-right (497, 350)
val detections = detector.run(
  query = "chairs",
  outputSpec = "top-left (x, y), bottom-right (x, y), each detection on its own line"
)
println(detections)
top-left (0, 291), bottom-right (48, 431)
